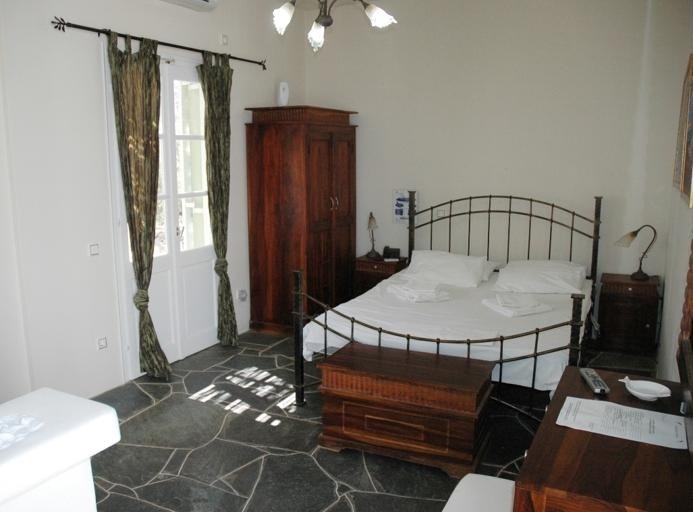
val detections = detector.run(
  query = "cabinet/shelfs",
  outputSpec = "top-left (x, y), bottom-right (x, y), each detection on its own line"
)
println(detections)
top-left (244, 107), bottom-right (359, 337)
top-left (513, 365), bottom-right (693, 511)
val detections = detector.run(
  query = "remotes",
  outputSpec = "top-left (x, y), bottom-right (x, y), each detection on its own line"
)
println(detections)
top-left (579, 367), bottom-right (610, 395)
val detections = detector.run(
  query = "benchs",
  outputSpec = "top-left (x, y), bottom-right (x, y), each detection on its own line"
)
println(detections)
top-left (316, 342), bottom-right (493, 479)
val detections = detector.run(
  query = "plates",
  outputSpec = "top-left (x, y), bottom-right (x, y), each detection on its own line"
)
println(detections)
top-left (617, 375), bottom-right (672, 402)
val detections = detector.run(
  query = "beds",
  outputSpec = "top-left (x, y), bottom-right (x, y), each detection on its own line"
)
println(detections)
top-left (285, 183), bottom-right (605, 419)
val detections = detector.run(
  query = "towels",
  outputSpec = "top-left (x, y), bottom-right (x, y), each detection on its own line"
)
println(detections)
top-left (387, 278), bottom-right (554, 319)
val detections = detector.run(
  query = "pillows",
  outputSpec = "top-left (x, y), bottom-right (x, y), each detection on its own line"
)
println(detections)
top-left (491, 258), bottom-right (584, 289)
top-left (408, 249), bottom-right (481, 287)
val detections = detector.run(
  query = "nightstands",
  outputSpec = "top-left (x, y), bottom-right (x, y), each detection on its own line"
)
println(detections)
top-left (598, 270), bottom-right (660, 358)
top-left (353, 256), bottom-right (404, 293)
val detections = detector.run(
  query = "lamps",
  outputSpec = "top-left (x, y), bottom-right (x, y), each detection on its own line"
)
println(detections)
top-left (367, 213), bottom-right (382, 260)
top-left (616, 226), bottom-right (664, 280)
top-left (266, 0), bottom-right (399, 54)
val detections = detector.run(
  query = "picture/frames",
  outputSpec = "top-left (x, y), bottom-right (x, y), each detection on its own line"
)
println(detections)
top-left (678, 52), bottom-right (692, 208)
